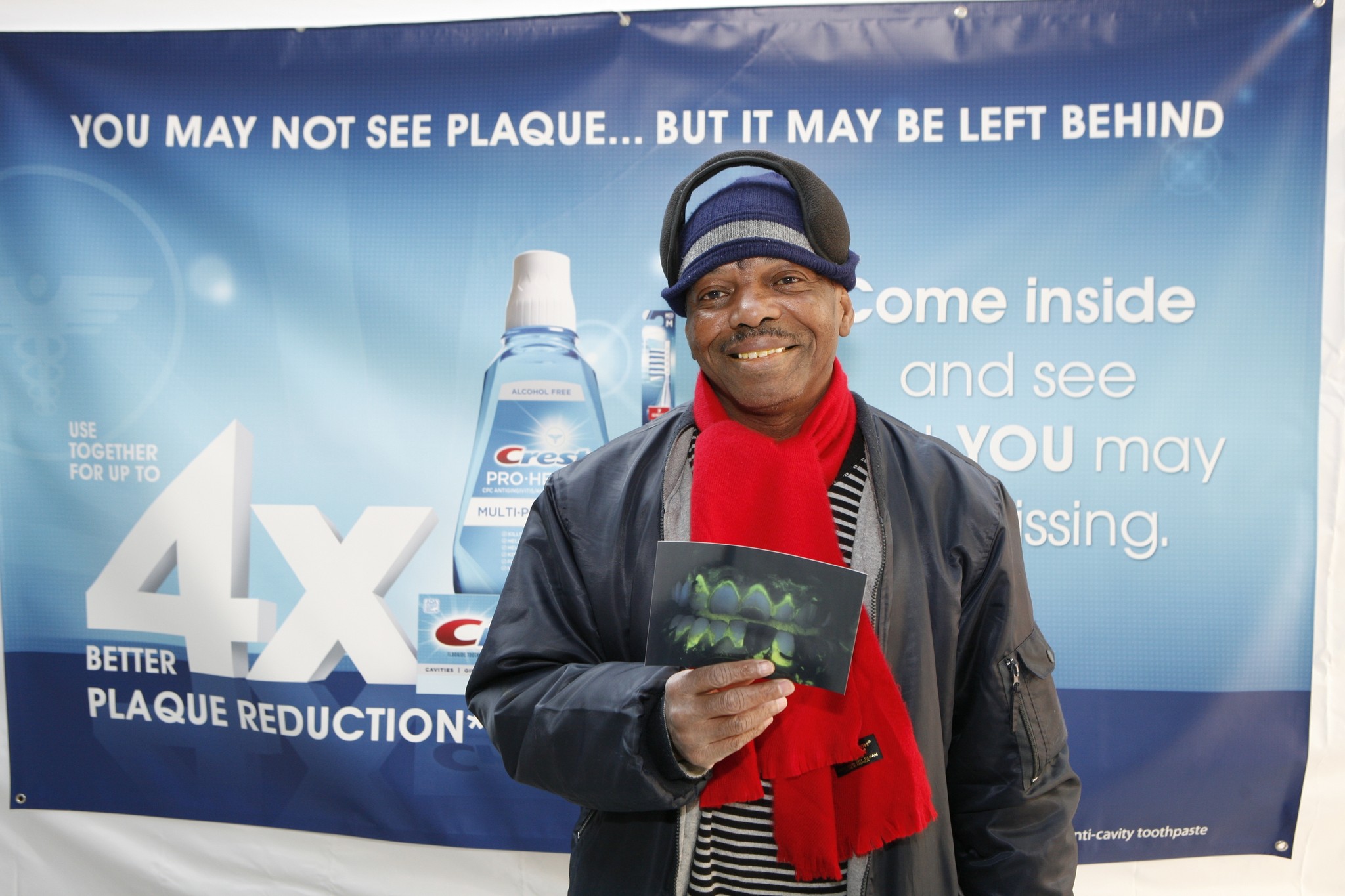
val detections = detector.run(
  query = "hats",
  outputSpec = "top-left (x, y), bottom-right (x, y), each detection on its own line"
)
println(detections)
top-left (663, 172), bottom-right (857, 317)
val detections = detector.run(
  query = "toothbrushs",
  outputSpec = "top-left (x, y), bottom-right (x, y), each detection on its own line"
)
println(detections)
top-left (640, 325), bottom-right (681, 428)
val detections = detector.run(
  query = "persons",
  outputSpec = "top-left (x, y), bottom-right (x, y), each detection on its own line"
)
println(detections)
top-left (462, 147), bottom-right (1086, 896)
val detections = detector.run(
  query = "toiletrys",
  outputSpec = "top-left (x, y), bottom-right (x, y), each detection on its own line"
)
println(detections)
top-left (413, 596), bottom-right (513, 696)
top-left (446, 246), bottom-right (615, 594)
